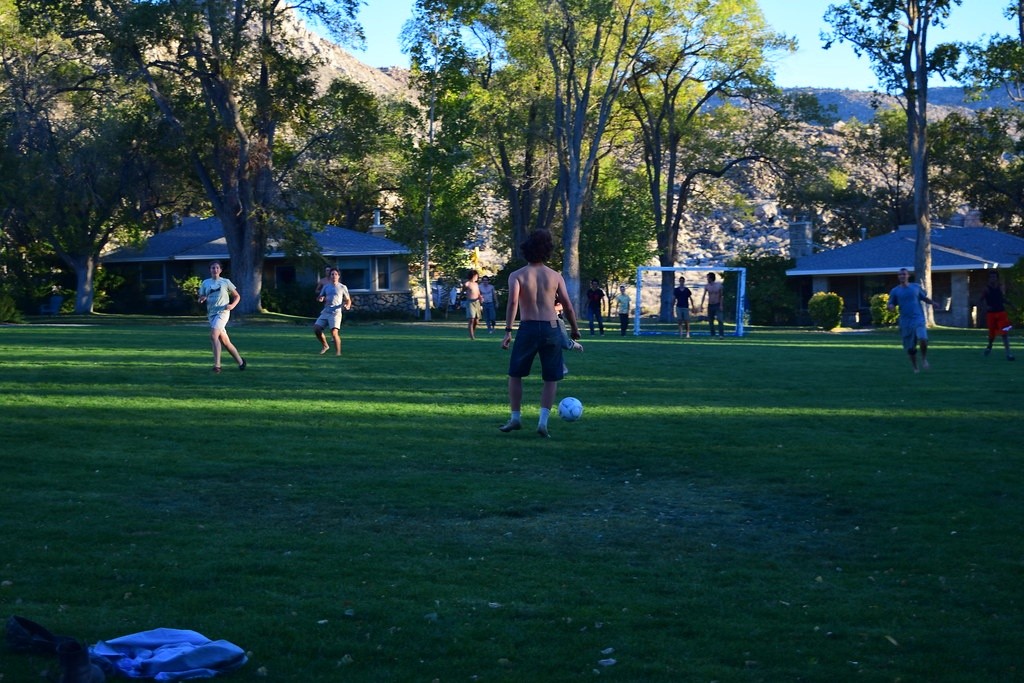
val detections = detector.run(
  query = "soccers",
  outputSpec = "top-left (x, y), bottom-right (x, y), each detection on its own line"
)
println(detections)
top-left (556, 396), bottom-right (585, 422)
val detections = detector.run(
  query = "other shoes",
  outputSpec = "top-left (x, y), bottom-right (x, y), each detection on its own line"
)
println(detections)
top-left (499, 419), bottom-right (522, 432)
top-left (336, 353), bottom-right (341, 357)
top-left (239, 357), bottom-right (246, 371)
top-left (537, 424), bottom-right (552, 438)
top-left (320, 346), bottom-right (329, 355)
top-left (213, 367), bottom-right (220, 373)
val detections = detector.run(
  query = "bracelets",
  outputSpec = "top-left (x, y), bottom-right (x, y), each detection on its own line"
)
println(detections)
top-left (505, 327), bottom-right (512, 332)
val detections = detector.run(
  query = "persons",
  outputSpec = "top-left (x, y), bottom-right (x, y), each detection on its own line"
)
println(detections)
top-left (456, 270), bottom-right (481, 340)
top-left (479, 275), bottom-right (499, 335)
top-left (497, 229), bottom-right (582, 440)
top-left (314, 266), bottom-right (351, 356)
top-left (585, 279), bottom-right (605, 338)
top-left (701, 271), bottom-right (724, 337)
top-left (197, 262), bottom-right (246, 373)
top-left (977, 272), bottom-right (1015, 361)
top-left (888, 268), bottom-right (940, 374)
top-left (673, 276), bottom-right (695, 338)
top-left (615, 285), bottom-right (631, 336)
